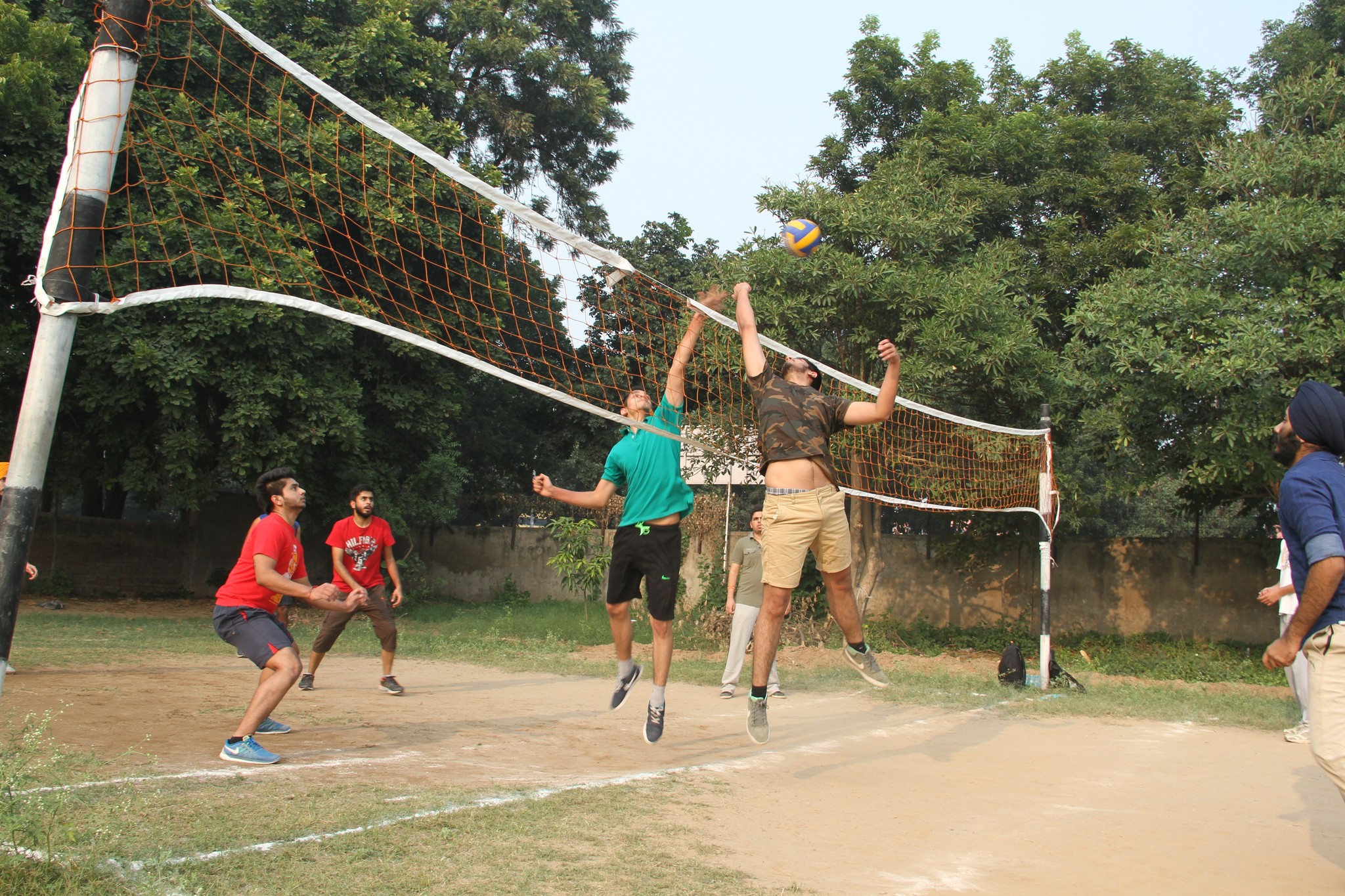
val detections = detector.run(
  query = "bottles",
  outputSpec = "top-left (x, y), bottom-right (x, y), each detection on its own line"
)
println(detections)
top-left (1025, 674), bottom-right (1029, 684)
top-left (1042, 694), bottom-right (1066, 700)
top-left (1034, 675), bottom-right (1040, 687)
top-left (1029, 675), bottom-right (1034, 685)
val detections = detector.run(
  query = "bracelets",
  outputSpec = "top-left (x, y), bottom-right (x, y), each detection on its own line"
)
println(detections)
top-left (308, 585), bottom-right (319, 601)
top-left (727, 586), bottom-right (736, 590)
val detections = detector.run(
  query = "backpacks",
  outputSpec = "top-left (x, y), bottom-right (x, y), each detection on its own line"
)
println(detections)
top-left (1048, 660), bottom-right (1087, 694)
top-left (997, 645), bottom-right (1027, 689)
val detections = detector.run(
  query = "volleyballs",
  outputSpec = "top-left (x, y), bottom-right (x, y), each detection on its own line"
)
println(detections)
top-left (782, 219), bottom-right (821, 257)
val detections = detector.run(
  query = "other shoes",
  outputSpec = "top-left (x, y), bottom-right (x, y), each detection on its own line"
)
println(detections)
top-left (6, 663), bottom-right (16, 675)
top-left (771, 691), bottom-right (786, 698)
top-left (720, 691), bottom-right (731, 698)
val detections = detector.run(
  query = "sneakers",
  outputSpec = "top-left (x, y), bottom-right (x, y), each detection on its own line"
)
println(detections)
top-left (379, 676), bottom-right (404, 694)
top-left (219, 733), bottom-right (280, 764)
top-left (643, 696), bottom-right (665, 745)
top-left (746, 687), bottom-right (770, 744)
top-left (610, 664), bottom-right (644, 712)
top-left (841, 642), bottom-right (890, 687)
top-left (1283, 721), bottom-right (1310, 744)
top-left (254, 717), bottom-right (291, 735)
top-left (298, 674), bottom-right (315, 690)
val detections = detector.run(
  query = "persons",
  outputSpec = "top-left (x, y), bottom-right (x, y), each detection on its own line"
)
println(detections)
top-left (531, 283), bottom-right (728, 745)
top-left (297, 484), bottom-right (404, 694)
top-left (732, 281), bottom-right (900, 745)
top-left (1255, 379), bottom-right (1345, 801)
top-left (212, 468), bottom-right (364, 764)
top-left (719, 506), bottom-right (786, 698)
top-left (0, 462), bottom-right (38, 674)
